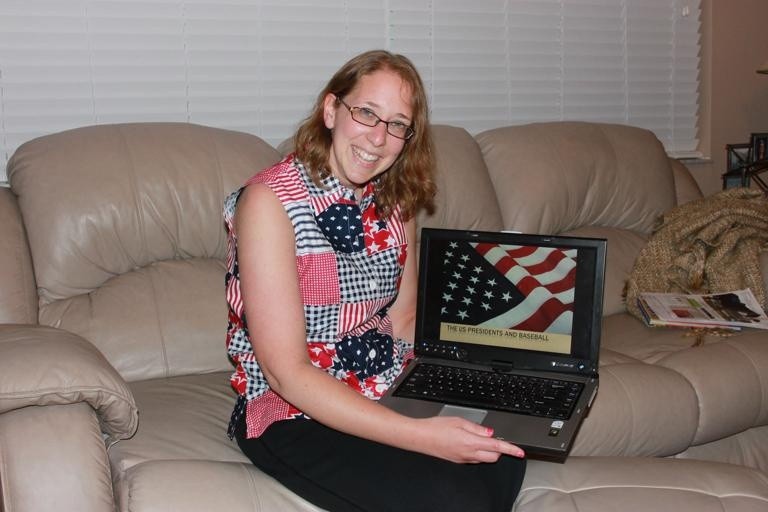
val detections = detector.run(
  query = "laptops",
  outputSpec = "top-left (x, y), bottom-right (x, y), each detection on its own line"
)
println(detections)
top-left (377, 228), bottom-right (607, 465)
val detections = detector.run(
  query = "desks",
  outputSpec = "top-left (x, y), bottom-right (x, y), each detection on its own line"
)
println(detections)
top-left (721, 133), bottom-right (768, 193)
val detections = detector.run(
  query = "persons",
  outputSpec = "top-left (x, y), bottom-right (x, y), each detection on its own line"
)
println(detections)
top-left (221, 50), bottom-right (528, 512)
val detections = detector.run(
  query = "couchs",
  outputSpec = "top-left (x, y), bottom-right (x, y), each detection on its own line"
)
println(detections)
top-left (0, 120), bottom-right (767, 512)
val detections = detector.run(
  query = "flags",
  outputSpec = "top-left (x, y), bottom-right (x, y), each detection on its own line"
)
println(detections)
top-left (437, 237), bottom-right (578, 356)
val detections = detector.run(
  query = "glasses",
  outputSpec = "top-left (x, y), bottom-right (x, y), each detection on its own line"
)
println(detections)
top-left (336, 92), bottom-right (416, 144)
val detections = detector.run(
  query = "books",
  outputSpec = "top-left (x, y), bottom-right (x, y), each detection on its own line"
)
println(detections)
top-left (638, 285), bottom-right (768, 332)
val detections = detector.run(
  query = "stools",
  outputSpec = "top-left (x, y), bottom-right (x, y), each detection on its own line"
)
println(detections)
top-left (511, 454), bottom-right (768, 512)
